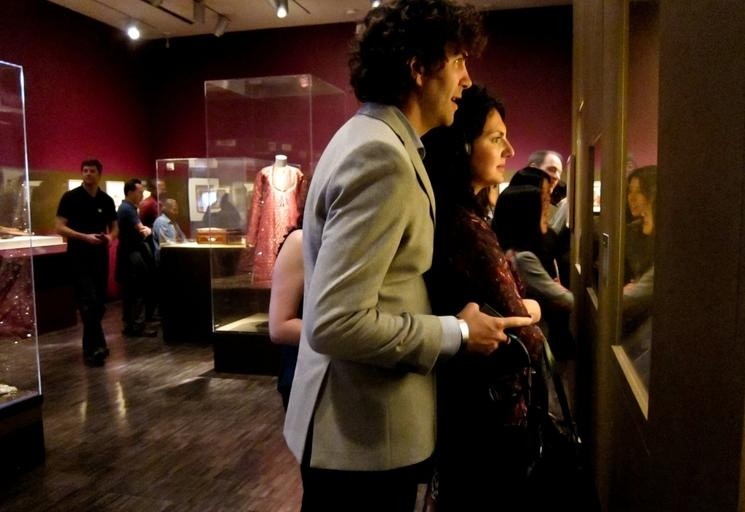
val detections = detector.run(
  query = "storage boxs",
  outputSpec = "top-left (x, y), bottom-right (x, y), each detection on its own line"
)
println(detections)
top-left (194, 226), bottom-right (243, 246)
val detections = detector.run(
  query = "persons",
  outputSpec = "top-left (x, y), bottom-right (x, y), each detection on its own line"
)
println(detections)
top-left (201, 190), bottom-right (240, 230)
top-left (116, 178), bottom-right (157, 338)
top-left (246, 154), bottom-right (305, 285)
top-left (53, 159), bottom-right (120, 367)
top-left (138, 180), bottom-right (168, 321)
top-left (621, 156), bottom-right (658, 391)
top-left (153, 198), bottom-right (188, 262)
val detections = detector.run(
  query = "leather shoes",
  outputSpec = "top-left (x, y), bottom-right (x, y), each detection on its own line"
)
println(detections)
top-left (83, 345), bottom-right (110, 368)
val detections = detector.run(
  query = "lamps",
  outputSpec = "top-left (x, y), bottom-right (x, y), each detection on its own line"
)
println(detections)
top-left (126, 17), bottom-right (146, 40)
top-left (190, 2), bottom-right (231, 38)
top-left (275, 1), bottom-right (290, 19)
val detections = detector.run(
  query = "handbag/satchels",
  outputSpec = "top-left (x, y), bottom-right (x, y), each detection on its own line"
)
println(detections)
top-left (428, 390), bottom-right (602, 512)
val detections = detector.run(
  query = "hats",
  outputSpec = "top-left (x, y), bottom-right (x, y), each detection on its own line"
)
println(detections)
top-left (528, 151), bottom-right (565, 180)
